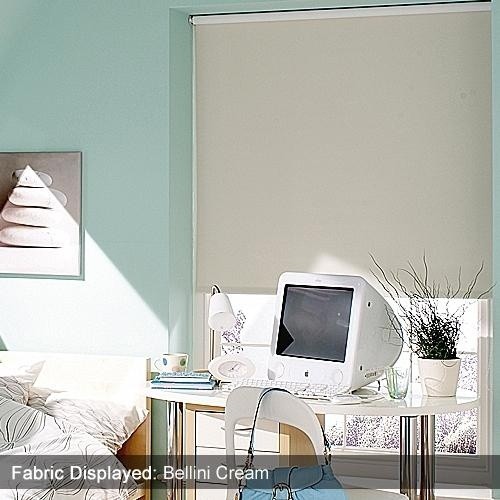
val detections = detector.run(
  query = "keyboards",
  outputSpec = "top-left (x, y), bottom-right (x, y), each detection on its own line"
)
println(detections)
top-left (236, 375), bottom-right (350, 400)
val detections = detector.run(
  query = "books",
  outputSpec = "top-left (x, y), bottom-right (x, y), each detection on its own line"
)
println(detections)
top-left (158, 371), bottom-right (212, 383)
top-left (152, 376), bottom-right (217, 391)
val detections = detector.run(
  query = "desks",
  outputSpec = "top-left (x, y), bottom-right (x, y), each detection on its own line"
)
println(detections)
top-left (147, 365), bottom-right (480, 499)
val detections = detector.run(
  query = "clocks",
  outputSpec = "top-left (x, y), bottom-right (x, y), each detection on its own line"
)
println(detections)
top-left (207, 354), bottom-right (256, 383)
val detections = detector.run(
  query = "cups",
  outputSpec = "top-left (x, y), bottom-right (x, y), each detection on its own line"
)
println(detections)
top-left (384, 367), bottom-right (409, 400)
top-left (154, 352), bottom-right (189, 373)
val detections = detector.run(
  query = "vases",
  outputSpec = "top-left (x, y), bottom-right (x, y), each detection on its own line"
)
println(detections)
top-left (416, 356), bottom-right (462, 399)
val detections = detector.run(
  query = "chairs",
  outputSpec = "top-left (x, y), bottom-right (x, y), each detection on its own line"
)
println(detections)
top-left (220, 386), bottom-right (412, 500)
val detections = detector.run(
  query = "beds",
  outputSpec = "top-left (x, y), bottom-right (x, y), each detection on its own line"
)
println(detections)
top-left (1, 350), bottom-right (157, 500)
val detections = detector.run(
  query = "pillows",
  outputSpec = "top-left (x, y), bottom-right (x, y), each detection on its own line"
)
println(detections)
top-left (1, 358), bottom-right (45, 406)
top-left (27, 384), bottom-right (152, 458)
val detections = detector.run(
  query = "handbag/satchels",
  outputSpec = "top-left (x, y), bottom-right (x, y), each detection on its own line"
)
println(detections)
top-left (236, 387), bottom-right (348, 500)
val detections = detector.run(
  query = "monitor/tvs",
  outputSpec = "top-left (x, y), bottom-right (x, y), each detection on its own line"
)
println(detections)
top-left (266, 271), bottom-right (403, 394)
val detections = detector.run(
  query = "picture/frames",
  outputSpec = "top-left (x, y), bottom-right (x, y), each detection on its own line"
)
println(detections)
top-left (0, 149), bottom-right (86, 282)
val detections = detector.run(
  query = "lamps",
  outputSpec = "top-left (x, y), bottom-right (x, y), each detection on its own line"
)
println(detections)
top-left (206, 285), bottom-right (239, 363)
